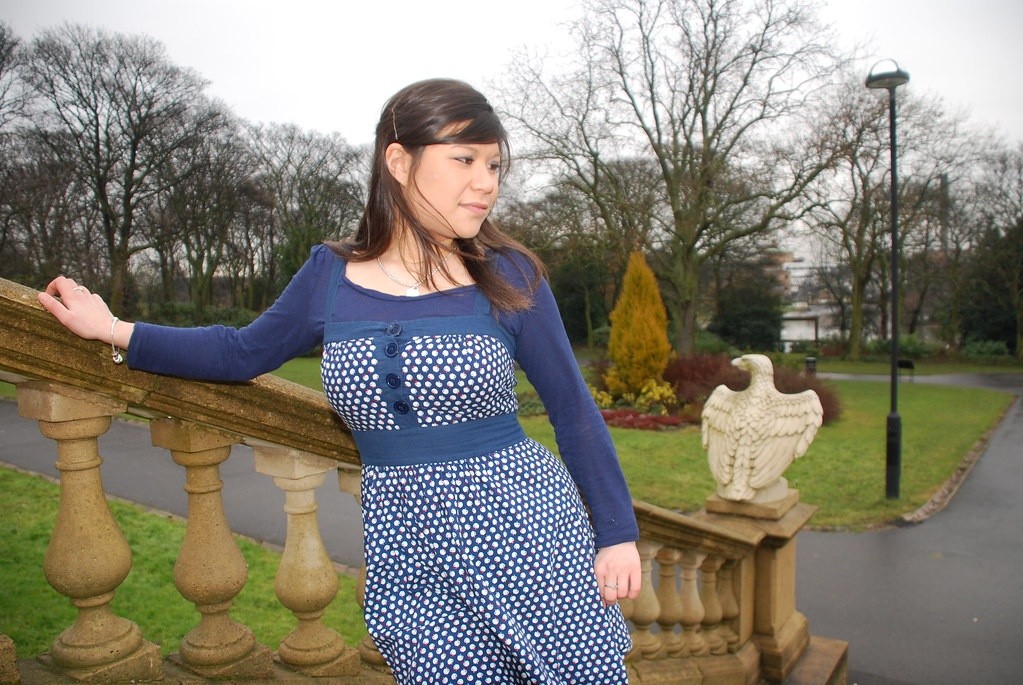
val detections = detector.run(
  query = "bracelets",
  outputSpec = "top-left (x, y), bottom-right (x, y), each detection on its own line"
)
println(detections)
top-left (111, 317), bottom-right (123, 364)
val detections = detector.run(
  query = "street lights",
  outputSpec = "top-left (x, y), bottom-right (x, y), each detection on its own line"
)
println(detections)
top-left (865, 58), bottom-right (909, 502)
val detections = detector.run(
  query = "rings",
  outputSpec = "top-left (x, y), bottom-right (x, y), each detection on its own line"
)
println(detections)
top-left (72, 286), bottom-right (83, 291)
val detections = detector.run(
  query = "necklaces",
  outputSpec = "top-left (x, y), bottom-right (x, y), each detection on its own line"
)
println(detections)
top-left (377, 242), bottom-right (457, 298)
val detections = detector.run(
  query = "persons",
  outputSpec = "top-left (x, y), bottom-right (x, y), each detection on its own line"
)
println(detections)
top-left (38, 77), bottom-right (643, 685)
